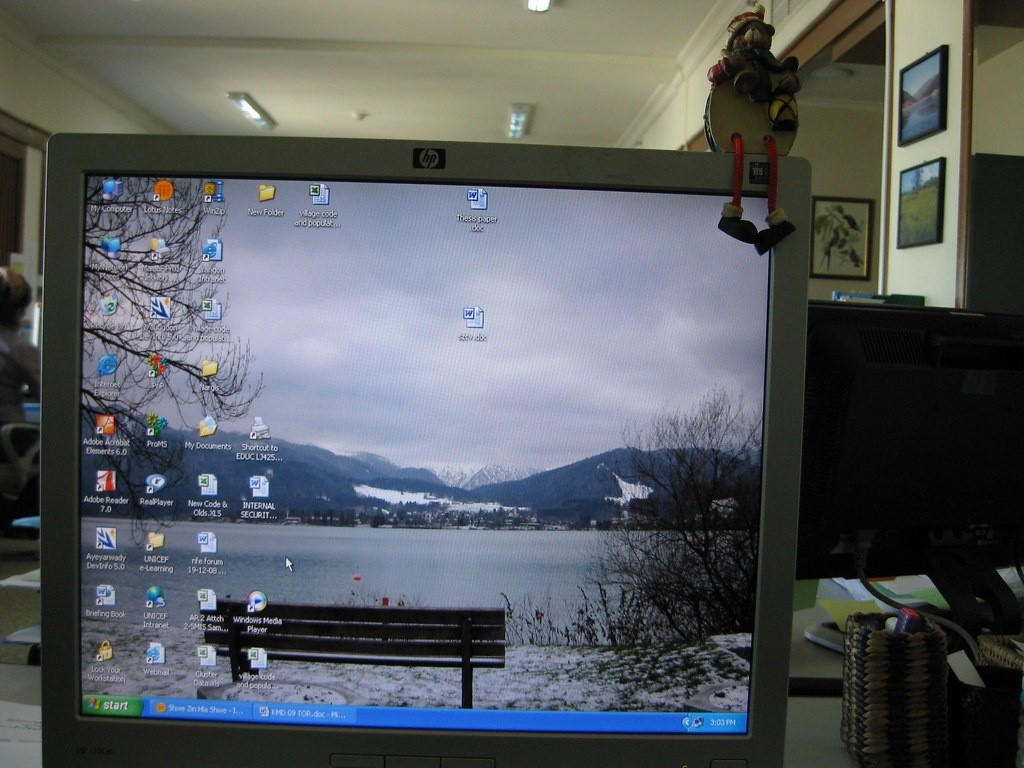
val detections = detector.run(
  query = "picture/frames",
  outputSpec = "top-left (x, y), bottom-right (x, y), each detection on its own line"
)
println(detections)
top-left (898, 44), bottom-right (948, 147)
top-left (897, 158), bottom-right (946, 249)
top-left (809, 196), bottom-right (875, 281)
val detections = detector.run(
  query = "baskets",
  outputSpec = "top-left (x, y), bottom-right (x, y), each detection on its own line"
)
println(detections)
top-left (976, 634), bottom-right (1024, 672)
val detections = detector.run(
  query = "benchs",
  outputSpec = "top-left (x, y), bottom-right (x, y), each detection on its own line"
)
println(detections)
top-left (201, 597), bottom-right (507, 708)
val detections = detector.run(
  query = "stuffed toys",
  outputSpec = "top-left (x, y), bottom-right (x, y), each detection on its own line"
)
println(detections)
top-left (708, 4), bottom-right (802, 102)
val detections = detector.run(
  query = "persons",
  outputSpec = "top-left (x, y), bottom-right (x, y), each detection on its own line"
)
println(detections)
top-left (0, 267), bottom-right (40, 424)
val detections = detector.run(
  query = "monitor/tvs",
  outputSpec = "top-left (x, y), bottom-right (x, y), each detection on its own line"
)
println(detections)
top-left (38, 132), bottom-right (812, 768)
top-left (805, 296), bottom-right (1024, 645)
